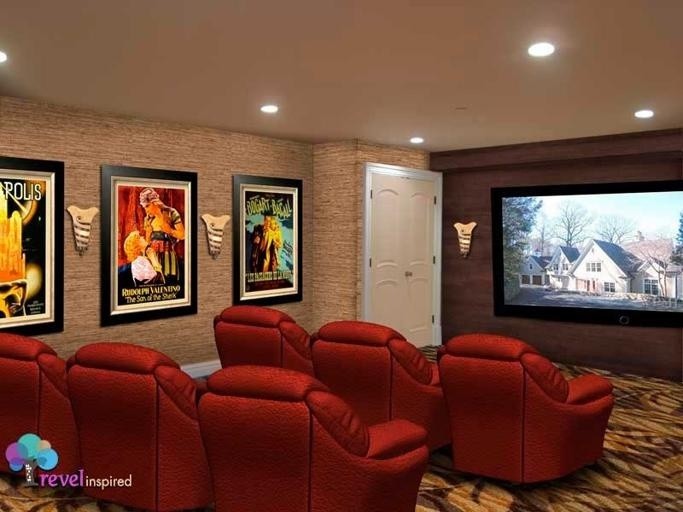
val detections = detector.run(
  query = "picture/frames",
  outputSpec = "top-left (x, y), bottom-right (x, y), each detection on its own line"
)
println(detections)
top-left (232, 174), bottom-right (302, 306)
top-left (100, 165), bottom-right (197, 326)
top-left (0, 156), bottom-right (64, 337)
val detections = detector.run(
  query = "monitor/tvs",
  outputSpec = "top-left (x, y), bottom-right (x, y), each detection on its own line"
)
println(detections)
top-left (491, 179), bottom-right (683, 329)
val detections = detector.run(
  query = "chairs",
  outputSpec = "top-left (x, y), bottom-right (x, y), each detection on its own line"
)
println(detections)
top-left (436, 332), bottom-right (615, 484)
top-left (308, 321), bottom-right (453, 452)
top-left (213, 305), bottom-right (314, 379)
top-left (65, 342), bottom-right (213, 512)
top-left (0, 333), bottom-right (79, 482)
top-left (194, 365), bottom-right (429, 512)
